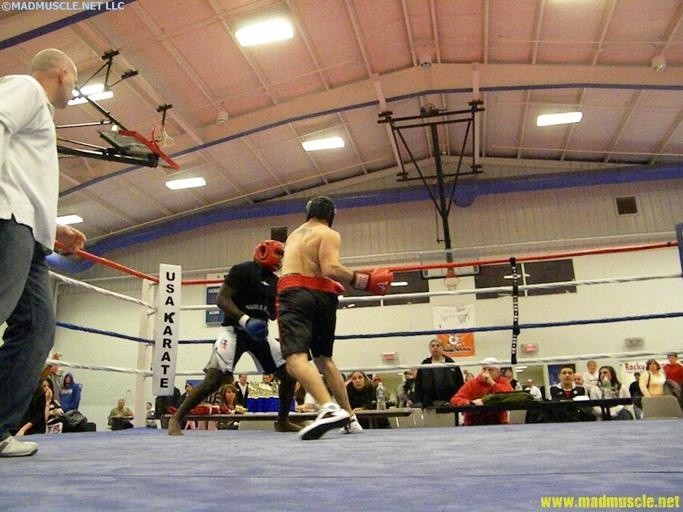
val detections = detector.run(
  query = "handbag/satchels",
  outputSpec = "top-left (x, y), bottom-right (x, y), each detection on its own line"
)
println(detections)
top-left (483, 390), bottom-right (533, 407)
top-left (635, 397), bottom-right (641, 408)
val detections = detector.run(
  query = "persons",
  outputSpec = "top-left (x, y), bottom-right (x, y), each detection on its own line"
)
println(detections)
top-left (272, 196), bottom-right (394, 441)
top-left (0, 48), bottom-right (88, 458)
top-left (11, 350), bottom-right (683, 440)
top-left (167, 239), bottom-right (304, 436)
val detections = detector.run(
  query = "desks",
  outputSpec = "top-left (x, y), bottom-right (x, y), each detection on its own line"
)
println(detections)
top-left (162, 409), bottom-right (415, 428)
top-left (432, 399), bottom-right (634, 426)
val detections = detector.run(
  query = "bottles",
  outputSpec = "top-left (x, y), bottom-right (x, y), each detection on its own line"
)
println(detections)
top-left (604, 377), bottom-right (613, 399)
top-left (375, 383), bottom-right (386, 410)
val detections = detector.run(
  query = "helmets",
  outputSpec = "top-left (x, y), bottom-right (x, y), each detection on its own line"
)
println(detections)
top-left (253, 239), bottom-right (285, 271)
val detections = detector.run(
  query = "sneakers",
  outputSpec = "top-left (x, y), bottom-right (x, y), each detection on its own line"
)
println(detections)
top-left (0, 435), bottom-right (37, 457)
top-left (299, 407), bottom-right (362, 440)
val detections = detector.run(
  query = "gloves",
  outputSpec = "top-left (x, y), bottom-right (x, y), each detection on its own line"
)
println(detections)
top-left (350, 267), bottom-right (394, 296)
top-left (239, 314), bottom-right (269, 341)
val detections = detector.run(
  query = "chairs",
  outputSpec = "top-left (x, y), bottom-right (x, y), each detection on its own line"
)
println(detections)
top-left (642, 395), bottom-right (680, 420)
